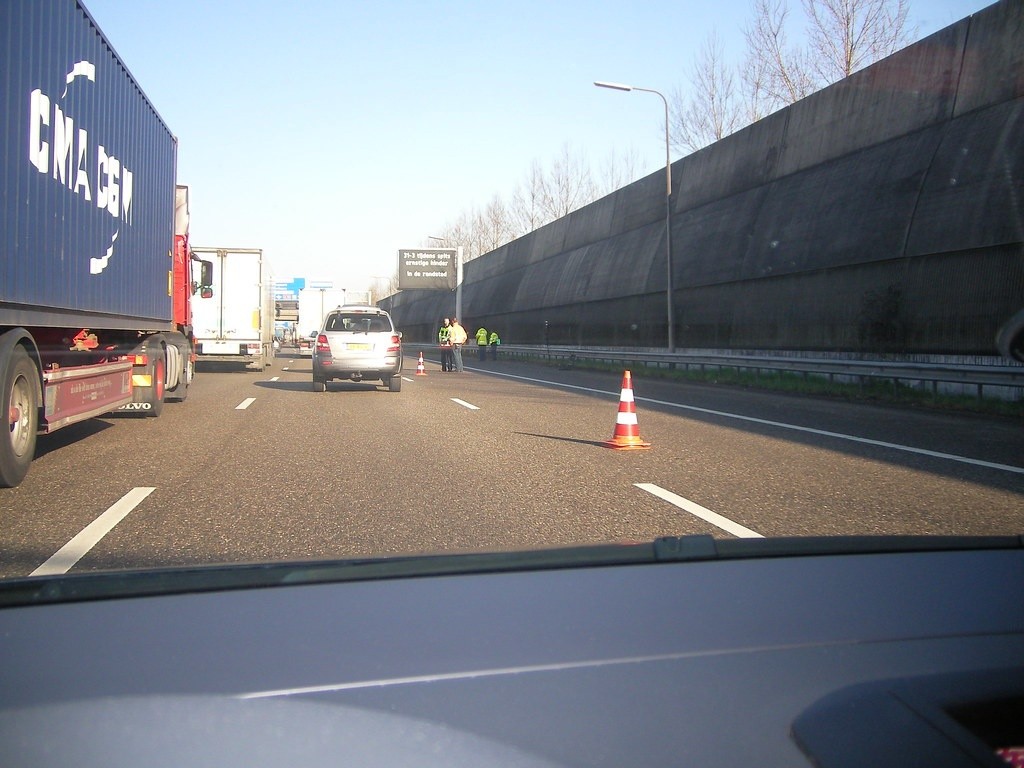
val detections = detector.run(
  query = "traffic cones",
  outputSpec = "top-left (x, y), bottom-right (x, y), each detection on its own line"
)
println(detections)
top-left (601, 370), bottom-right (651, 451)
top-left (415, 351), bottom-right (426, 375)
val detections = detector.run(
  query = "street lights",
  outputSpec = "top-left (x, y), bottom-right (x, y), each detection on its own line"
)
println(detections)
top-left (374, 276), bottom-right (392, 319)
top-left (342, 288), bottom-right (346, 306)
top-left (595, 82), bottom-right (675, 372)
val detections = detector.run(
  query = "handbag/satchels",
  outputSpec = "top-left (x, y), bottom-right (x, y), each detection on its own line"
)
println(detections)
top-left (496, 339), bottom-right (500, 345)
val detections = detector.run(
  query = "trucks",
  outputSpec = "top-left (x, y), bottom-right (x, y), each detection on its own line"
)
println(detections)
top-left (191, 247), bottom-right (292, 372)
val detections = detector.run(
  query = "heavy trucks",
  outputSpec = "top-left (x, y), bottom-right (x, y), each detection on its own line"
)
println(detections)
top-left (0, 0), bottom-right (213, 488)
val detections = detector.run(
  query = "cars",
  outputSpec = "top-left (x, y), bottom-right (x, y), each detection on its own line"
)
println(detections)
top-left (295, 337), bottom-right (316, 358)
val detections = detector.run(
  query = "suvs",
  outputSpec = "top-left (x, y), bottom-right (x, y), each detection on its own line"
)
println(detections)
top-left (312, 304), bottom-right (404, 392)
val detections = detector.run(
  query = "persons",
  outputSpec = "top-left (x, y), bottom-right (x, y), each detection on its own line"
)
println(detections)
top-left (475, 325), bottom-right (488, 361)
top-left (489, 329), bottom-right (498, 360)
top-left (438, 316), bottom-right (468, 372)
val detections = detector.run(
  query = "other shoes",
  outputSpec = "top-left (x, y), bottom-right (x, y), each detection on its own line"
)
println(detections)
top-left (448, 369), bottom-right (451, 372)
top-left (453, 370), bottom-right (460, 373)
top-left (439, 368), bottom-right (446, 371)
